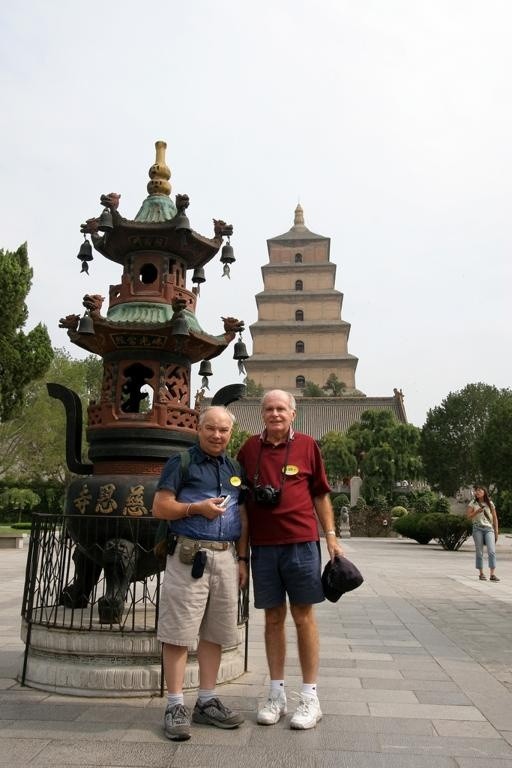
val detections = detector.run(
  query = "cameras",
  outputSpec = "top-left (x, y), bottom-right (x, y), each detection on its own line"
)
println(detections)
top-left (254, 486), bottom-right (280, 509)
top-left (216, 494), bottom-right (231, 508)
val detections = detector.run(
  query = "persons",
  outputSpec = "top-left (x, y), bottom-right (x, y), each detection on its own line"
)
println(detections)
top-left (234, 388), bottom-right (349, 731)
top-left (466, 485), bottom-right (502, 582)
top-left (145, 403), bottom-right (252, 742)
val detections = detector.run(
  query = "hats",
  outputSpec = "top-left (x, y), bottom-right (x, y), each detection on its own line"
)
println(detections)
top-left (322, 557), bottom-right (364, 602)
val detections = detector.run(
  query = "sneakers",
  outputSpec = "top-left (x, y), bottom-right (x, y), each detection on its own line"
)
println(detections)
top-left (479, 573), bottom-right (500, 581)
top-left (257, 692), bottom-right (288, 726)
top-left (164, 702), bottom-right (192, 740)
top-left (193, 698), bottom-right (245, 730)
top-left (289, 696), bottom-right (322, 731)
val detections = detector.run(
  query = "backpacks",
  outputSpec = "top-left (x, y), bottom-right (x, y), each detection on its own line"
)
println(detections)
top-left (154, 451), bottom-right (242, 562)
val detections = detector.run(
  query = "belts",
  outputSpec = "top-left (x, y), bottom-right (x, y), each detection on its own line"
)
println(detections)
top-left (177, 537), bottom-right (231, 550)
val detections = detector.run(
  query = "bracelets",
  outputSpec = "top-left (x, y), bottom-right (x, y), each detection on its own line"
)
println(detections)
top-left (325, 531), bottom-right (336, 536)
top-left (185, 502), bottom-right (196, 518)
top-left (237, 556), bottom-right (249, 564)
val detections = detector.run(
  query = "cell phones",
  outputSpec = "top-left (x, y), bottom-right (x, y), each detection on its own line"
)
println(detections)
top-left (481, 506), bottom-right (485, 509)
